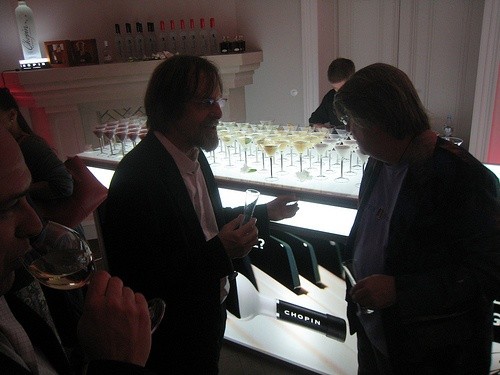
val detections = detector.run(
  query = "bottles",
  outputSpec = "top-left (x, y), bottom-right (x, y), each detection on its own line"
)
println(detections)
top-left (178, 19), bottom-right (190, 56)
top-left (168, 19), bottom-right (178, 54)
top-left (158, 21), bottom-right (169, 55)
top-left (209, 17), bottom-right (219, 54)
top-left (227, 187), bottom-right (261, 258)
top-left (103, 40), bottom-right (112, 64)
top-left (134, 22), bottom-right (148, 60)
top-left (125, 23), bottom-right (136, 59)
top-left (187, 19), bottom-right (199, 57)
top-left (198, 18), bottom-right (211, 56)
top-left (114, 23), bottom-right (127, 62)
top-left (148, 22), bottom-right (157, 57)
top-left (220, 33), bottom-right (247, 54)
top-left (13, 0), bottom-right (42, 61)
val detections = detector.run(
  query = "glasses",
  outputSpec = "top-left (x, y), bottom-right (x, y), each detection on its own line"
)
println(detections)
top-left (339, 114), bottom-right (351, 125)
top-left (192, 97), bottom-right (228, 107)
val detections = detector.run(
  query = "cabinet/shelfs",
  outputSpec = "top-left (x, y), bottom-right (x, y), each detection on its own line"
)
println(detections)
top-left (76, 142), bottom-right (500, 375)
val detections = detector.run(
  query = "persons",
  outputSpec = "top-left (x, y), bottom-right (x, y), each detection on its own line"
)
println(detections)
top-left (309, 58), bottom-right (355, 129)
top-left (0, 87), bottom-right (152, 375)
top-left (104, 55), bottom-right (302, 375)
top-left (332, 63), bottom-right (500, 375)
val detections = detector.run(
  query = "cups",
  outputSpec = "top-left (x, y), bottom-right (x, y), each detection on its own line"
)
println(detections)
top-left (342, 251), bottom-right (380, 314)
top-left (434, 131), bottom-right (463, 147)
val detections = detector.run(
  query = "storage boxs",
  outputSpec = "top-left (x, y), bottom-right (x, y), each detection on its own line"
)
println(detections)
top-left (44, 39), bottom-right (99, 67)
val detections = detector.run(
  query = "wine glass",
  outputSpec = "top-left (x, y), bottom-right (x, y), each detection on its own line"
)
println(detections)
top-left (203, 119), bottom-right (371, 192)
top-left (94, 114), bottom-right (150, 158)
top-left (20, 220), bottom-right (167, 334)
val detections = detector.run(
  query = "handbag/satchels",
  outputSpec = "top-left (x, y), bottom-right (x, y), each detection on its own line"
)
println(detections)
top-left (63, 155), bottom-right (108, 230)
